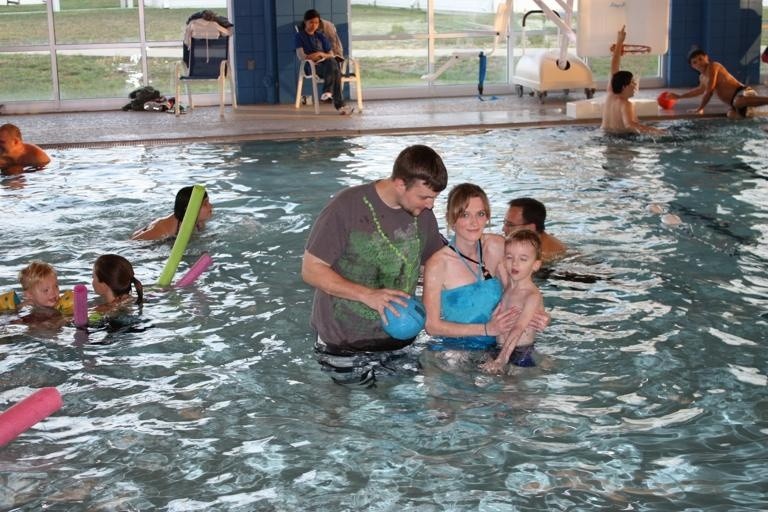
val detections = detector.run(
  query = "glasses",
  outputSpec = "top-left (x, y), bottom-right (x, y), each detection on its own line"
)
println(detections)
top-left (502, 220), bottom-right (526, 228)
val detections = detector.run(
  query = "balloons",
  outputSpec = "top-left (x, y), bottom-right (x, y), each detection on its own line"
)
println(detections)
top-left (657, 90), bottom-right (677, 110)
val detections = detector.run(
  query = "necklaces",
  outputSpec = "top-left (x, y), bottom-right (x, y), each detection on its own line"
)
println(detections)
top-left (451, 244), bottom-right (493, 281)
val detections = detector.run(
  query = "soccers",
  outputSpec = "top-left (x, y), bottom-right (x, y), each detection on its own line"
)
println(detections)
top-left (657, 92), bottom-right (676, 109)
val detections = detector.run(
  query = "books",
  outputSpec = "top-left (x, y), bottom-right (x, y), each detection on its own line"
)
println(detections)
top-left (314, 55), bottom-right (346, 65)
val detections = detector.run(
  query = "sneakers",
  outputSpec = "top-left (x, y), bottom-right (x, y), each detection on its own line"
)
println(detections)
top-left (338, 105), bottom-right (354, 115)
top-left (320, 92), bottom-right (332, 104)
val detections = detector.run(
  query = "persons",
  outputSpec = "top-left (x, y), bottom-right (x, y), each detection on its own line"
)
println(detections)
top-left (129, 185), bottom-right (215, 242)
top-left (478, 228), bottom-right (550, 378)
top-left (601, 70), bottom-right (658, 133)
top-left (10, 259), bottom-right (69, 324)
top-left (293, 8), bottom-right (347, 116)
top-left (79, 255), bottom-right (155, 336)
top-left (422, 182), bottom-right (509, 352)
top-left (665, 44), bottom-right (768, 119)
top-left (0, 122), bottom-right (51, 177)
top-left (300, 144), bottom-right (449, 392)
top-left (500, 197), bottom-right (571, 261)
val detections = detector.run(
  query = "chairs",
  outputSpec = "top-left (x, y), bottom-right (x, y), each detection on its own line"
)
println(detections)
top-left (294, 23), bottom-right (364, 114)
top-left (175, 34), bottom-right (237, 118)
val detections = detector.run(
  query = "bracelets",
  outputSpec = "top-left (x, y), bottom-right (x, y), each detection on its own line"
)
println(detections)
top-left (482, 321), bottom-right (490, 339)
